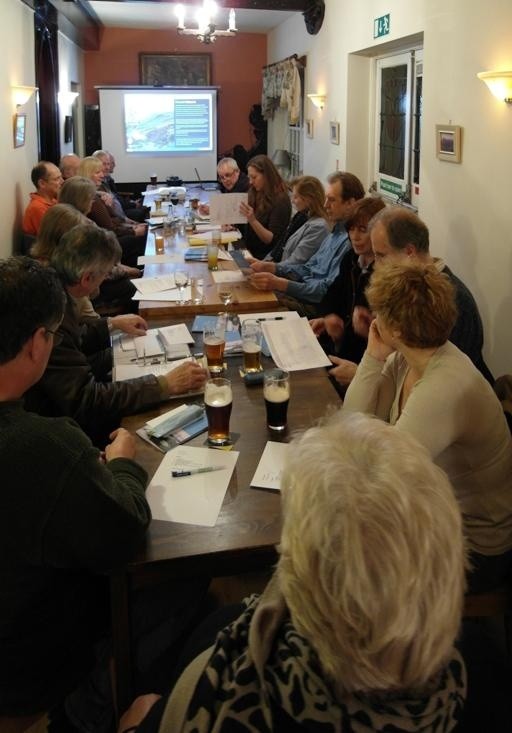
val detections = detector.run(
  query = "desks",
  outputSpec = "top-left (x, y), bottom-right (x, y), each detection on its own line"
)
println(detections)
top-left (129, 175), bottom-right (282, 320)
top-left (106, 311), bottom-right (343, 600)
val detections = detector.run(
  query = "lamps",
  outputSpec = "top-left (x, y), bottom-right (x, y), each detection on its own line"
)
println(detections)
top-left (474, 66), bottom-right (512, 106)
top-left (272, 147), bottom-right (292, 168)
top-left (305, 90), bottom-right (327, 112)
top-left (9, 80), bottom-right (39, 108)
top-left (172, 0), bottom-right (238, 42)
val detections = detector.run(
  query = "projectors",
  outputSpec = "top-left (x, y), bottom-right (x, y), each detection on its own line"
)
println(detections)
top-left (166, 175), bottom-right (183, 187)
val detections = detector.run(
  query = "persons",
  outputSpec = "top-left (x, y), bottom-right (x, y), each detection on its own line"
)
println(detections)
top-left (328, 204), bottom-right (498, 390)
top-left (18, 224), bottom-right (210, 451)
top-left (2, 255), bottom-right (165, 733)
top-left (341, 255), bottom-right (511, 597)
top-left (20, 148), bottom-right (148, 316)
top-left (202, 155), bottom-right (386, 339)
top-left (120, 403), bottom-right (468, 733)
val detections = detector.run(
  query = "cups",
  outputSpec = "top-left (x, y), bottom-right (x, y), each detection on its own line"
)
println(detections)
top-left (204, 376), bottom-right (234, 443)
top-left (190, 274), bottom-right (207, 304)
top-left (206, 242), bottom-right (221, 271)
top-left (240, 318), bottom-right (263, 372)
top-left (183, 212), bottom-right (195, 230)
top-left (210, 228), bottom-right (222, 245)
top-left (150, 175), bottom-right (158, 186)
top-left (155, 198), bottom-right (161, 208)
top-left (263, 368), bottom-right (290, 429)
top-left (202, 321), bottom-right (227, 372)
top-left (153, 229), bottom-right (165, 252)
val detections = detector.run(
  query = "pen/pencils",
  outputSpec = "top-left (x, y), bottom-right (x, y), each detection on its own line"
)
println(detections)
top-left (172, 466), bottom-right (226, 477)
top-left (258, 317), bottom-right (287, 321)
top-left (167, 353), bottom-right (204, 361)
top-left (130, 354), bottom-right (164, 361)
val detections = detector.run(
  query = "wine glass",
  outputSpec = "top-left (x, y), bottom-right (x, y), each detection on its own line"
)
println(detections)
top-left (174, 268), bottom-right (190, 305)
top-left (217, 281), bottom-right (233, 311)
top-left (170, 192), bottom-right (180, 205)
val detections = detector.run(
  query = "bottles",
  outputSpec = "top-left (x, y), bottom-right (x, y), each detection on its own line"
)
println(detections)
top-left (164, 204), bottom-right (177, 234)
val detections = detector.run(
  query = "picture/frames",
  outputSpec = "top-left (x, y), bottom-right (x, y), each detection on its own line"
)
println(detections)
top-left (434, 120), bottom-right (462, 163)
top-left (305, 118), bottom-right (313, 137)
top-left (13, 112), bottom-right (27, 149)
top-left (328, 120), bottom-right (340, 145)
top-left (136, 49), bottom-right (212, 87)
top-left (63, 114), bottom-right (75, 143)
